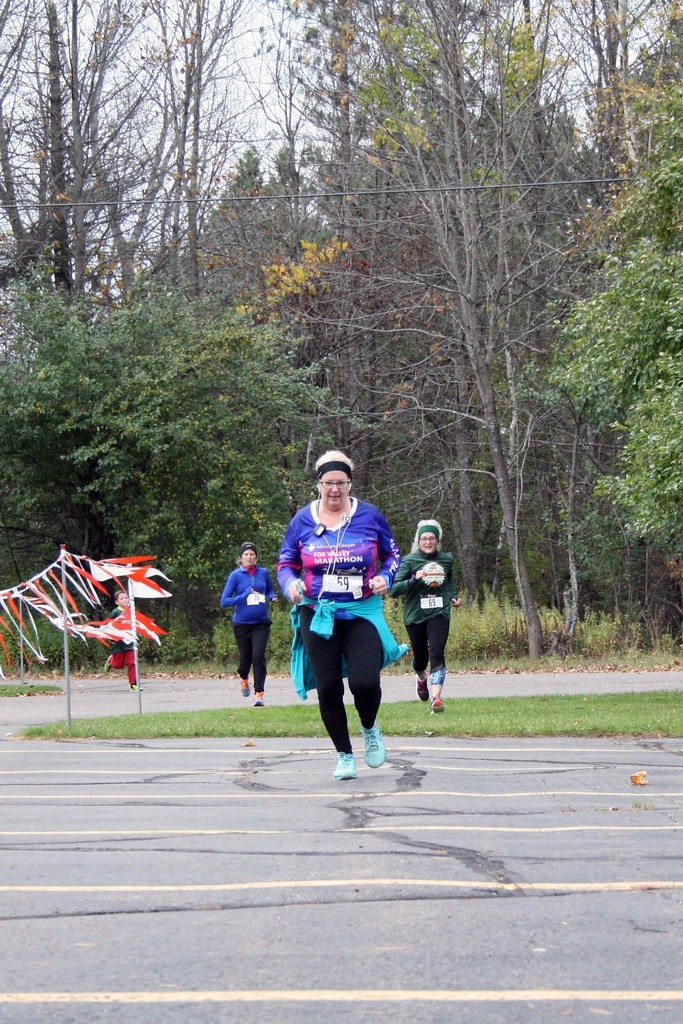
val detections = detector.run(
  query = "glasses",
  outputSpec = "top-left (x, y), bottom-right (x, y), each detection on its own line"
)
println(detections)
top-left (318, 479), bottom-right (351, 489)
top-left (419, 536), bottom-right (437, 543)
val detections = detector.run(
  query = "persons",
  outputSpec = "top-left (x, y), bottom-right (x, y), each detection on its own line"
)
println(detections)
top-left (389, 520), bottom-right (462, 714)
top-left (104, 590), bottom-right (142, 691)
top-left (277, 451), bottom-right (409, 781)
top-left (221, 543), bottom-right (280, 706)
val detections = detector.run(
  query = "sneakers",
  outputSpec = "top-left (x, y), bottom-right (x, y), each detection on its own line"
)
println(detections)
top-left (430, 699), bottom-right (444, 714)
top-left (334, 752), bottom-right (357, 780)
top-left (361, 726), bottom-right (385, 768)
top-left (253, 692), bottom-right (264, 707)
top-left (130, 684), bottom-right (144, 692)
top-left (240, 678), bottom-right (250, 697)
top-left (416, 673), bottom-right (429, 702)
top-left (104, 655), bottom-right (114, 672)
